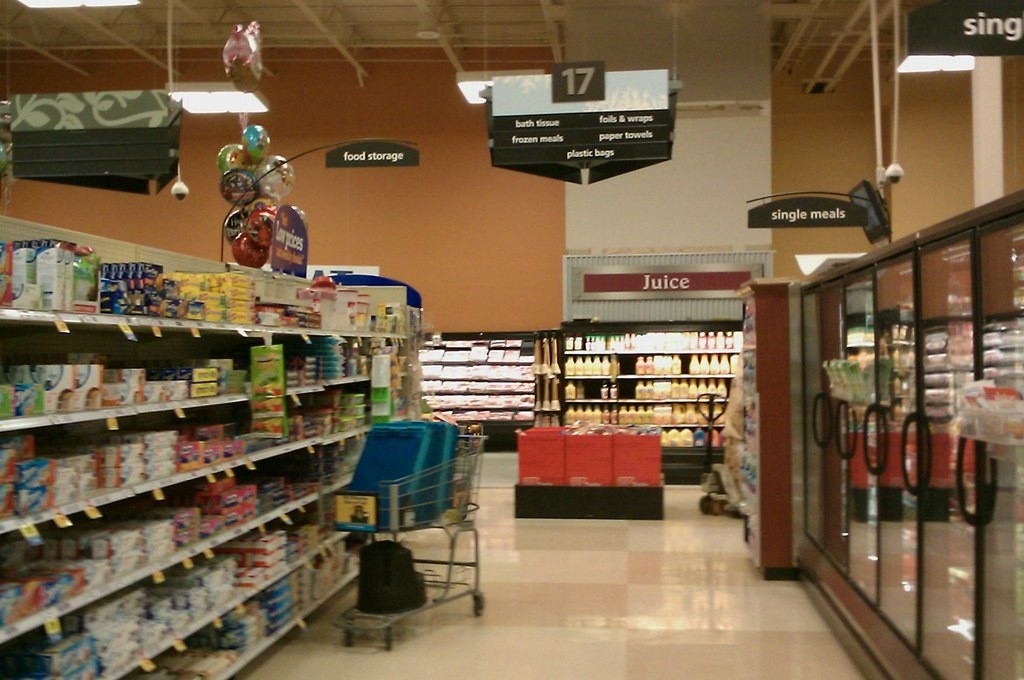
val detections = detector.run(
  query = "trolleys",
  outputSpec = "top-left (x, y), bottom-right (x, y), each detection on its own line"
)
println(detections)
top-left (695, 393), bottom-right (743, 519)
top-left (313, 420), bottom-right (487, 650)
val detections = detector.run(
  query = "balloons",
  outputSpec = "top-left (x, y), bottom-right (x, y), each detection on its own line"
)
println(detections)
top-left (222, 20), bottom-right (262, 93)
top-left (216, 124), bottom-right (295, 269)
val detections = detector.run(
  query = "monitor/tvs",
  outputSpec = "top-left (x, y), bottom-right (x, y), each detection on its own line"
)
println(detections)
top-left (848, 180), bottom-right (891, 243)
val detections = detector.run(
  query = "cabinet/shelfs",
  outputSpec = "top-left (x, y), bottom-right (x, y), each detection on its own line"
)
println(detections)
top-left (418, 331), bottom-right (539, 453)
top-left (531, 318), bottom-right (746, 483)
top-left (0, 212), bottom-right (429, 680)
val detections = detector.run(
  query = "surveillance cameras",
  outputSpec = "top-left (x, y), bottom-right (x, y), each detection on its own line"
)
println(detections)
top-left (171, 182), bottom-right (189, 200)
top-left (884, 163), bottom-right (905, 183)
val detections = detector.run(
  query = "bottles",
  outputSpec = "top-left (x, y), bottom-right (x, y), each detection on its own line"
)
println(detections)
top-left (730, 354), bottom-right (739, 373)
top-left (593, 356), bottom-right (601, 376)
top-left (661, 428), bottom-right (721, 447)
top-left (600, 384), bottom-right (609, 399)
top-left (565, 403), bottom-right (618, 423)
top-left (635, 378), bottom-right (727, 399)
top-left (566, 356), bottom-right (575, 375)
top-left (618, 404), bottom-right (724, 424)
top-left (635, 357), bottom-right (645, 375)
top-left (575, 356), bottom-right (583, 375)
top-left (710, 354), bottom-right (720, 374)
top-left (576, 380), bottom-right (585, 398)
top-left (700, 354), bottom-right (709, 374)
top-left (645, 357), bottom-right (653, 374)
top-left (720, 354), bottom-right (729, 374)
top-left (602, 355), bottom-right (611, 376)
top-left (610, 385), bottom-right (618, 399)
top-left (566, 333), bottom-right (605, 352)
top-left (689, 354), bottom-right (699, 374)
top-left (654, 355), bottom-right (682, 376)
top-left (583, 356), bottom-right (592, 376)
top-left (565, 380), bottom-right (576, 399)
top-left (612, 354), bottom-right (620, 376)
top-left (607, 332), bottom-right (734, 349)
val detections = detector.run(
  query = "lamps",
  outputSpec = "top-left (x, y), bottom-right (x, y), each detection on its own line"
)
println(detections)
top-left (455, 0), bottom-right (547, 104)
top-left (164, 0), bottom-right (274, 115)
top-left (884, 0), bottom-right (908, 183)
top-left (158, 1), bottom-right (189, 201)
top-left (663, 0), bottom-right (695, 101)
